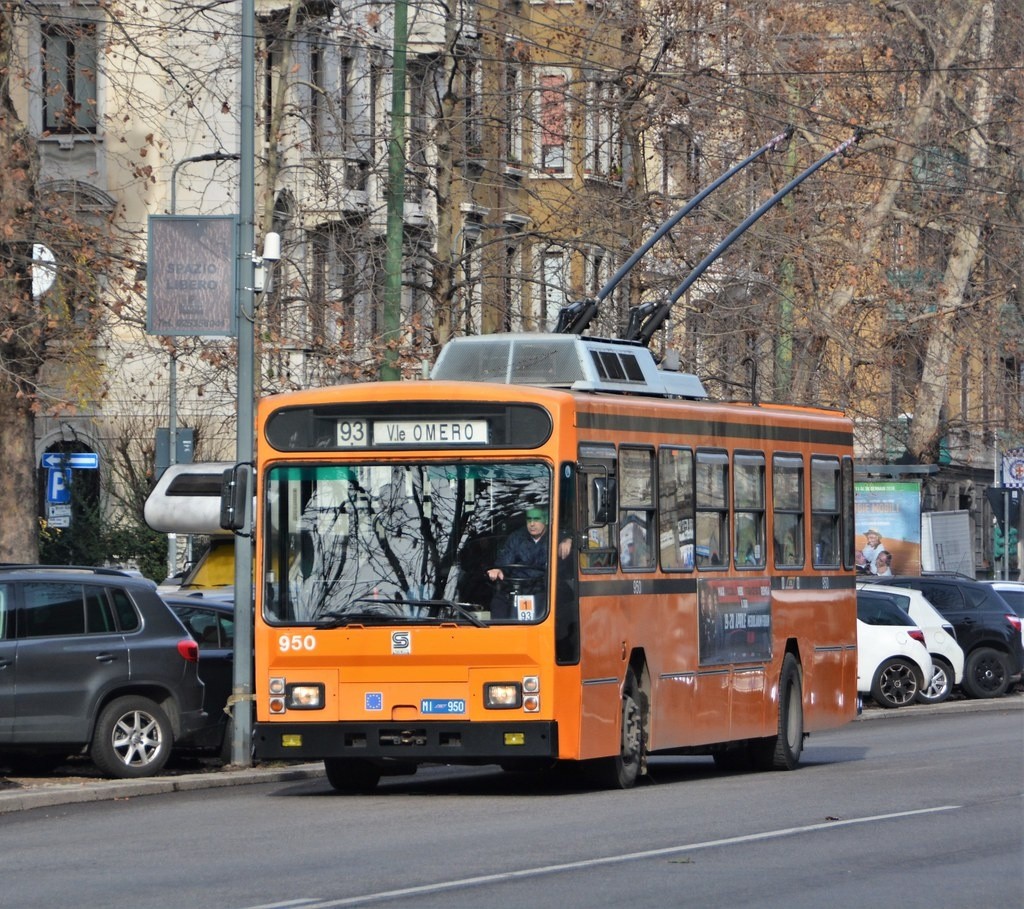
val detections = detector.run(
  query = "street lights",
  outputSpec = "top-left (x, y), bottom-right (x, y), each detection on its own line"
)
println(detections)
top-left (166, 150), bottom-right (239, 578)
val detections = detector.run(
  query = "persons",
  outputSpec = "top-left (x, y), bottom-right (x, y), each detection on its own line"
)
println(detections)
top-left (486, 503), bottom-right (574, 623)
top-left (856, 529), bottom-right (893, 576)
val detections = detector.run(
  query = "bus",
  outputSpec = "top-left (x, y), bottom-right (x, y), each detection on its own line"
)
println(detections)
top-left (219, 128), bottom-right (864, 797)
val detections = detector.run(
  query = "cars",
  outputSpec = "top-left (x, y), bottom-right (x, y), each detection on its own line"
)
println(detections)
top-left (157, 585), bottom-right (254, 752)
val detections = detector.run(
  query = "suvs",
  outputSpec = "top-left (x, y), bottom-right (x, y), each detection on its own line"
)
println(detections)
top-left (855, 570), bottom-right (1024, 709)
top-left (0, 562), bottom-right (208, 779)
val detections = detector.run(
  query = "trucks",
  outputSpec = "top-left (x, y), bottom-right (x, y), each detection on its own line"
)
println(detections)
top-left (144, 461), bottom-right (378, 591)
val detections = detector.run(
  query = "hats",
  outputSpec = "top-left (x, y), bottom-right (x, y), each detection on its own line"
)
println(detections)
top-left (863, 529), bottom-right (882, 540)
